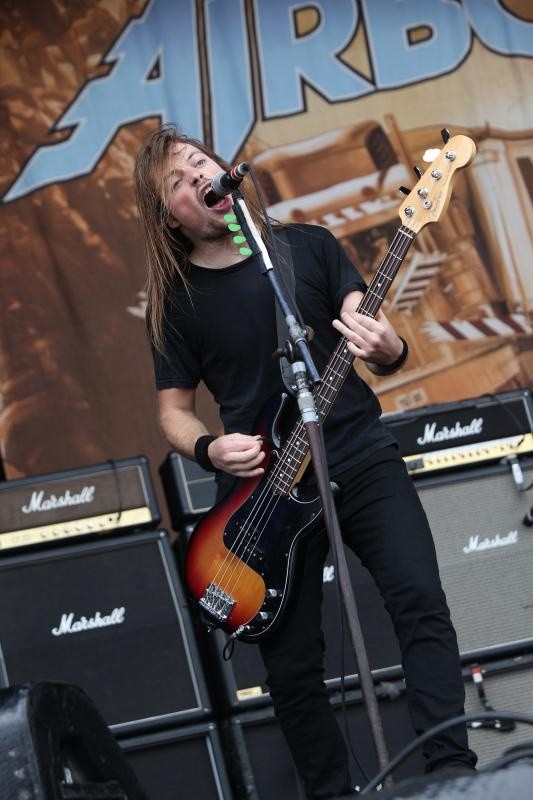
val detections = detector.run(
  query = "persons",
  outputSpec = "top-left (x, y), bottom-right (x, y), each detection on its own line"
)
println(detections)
top-left (133, 132), bottom-right (479, 800)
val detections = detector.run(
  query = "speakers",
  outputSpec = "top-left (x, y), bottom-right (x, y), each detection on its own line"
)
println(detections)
top-left (414, 458), bottom-right (533, 662)
top-left (463, 652), bottom-right (533, 770)
top-left (2, 678), bottom-right (150, 800)
top-left (117, 723), bottom-right (234, 800)
top-left (379, 388), bottom-right (533, 478)
top-left (1, 454), bottom-right (162, 555)
top-left (157, 450), bottom-right (218, 531)
top-left (0, 530), bottom-right (213, 742)
top-left (231, 680), bottom-right (426, 800)
top-left (214, 545), bottom-right (405, 706)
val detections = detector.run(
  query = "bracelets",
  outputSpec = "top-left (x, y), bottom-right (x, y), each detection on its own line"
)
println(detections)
top-left (194, 436), bottom-right (220, 473)
top-left (378, 335), bottom-right (408, 372)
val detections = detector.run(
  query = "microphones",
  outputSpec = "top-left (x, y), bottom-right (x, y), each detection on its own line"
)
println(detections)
top-left (208, 159), bottom-right (249, 199)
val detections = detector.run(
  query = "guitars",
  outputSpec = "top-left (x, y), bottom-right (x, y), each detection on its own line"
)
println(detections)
top-left (176, 133), bottom-right (478, 646)
